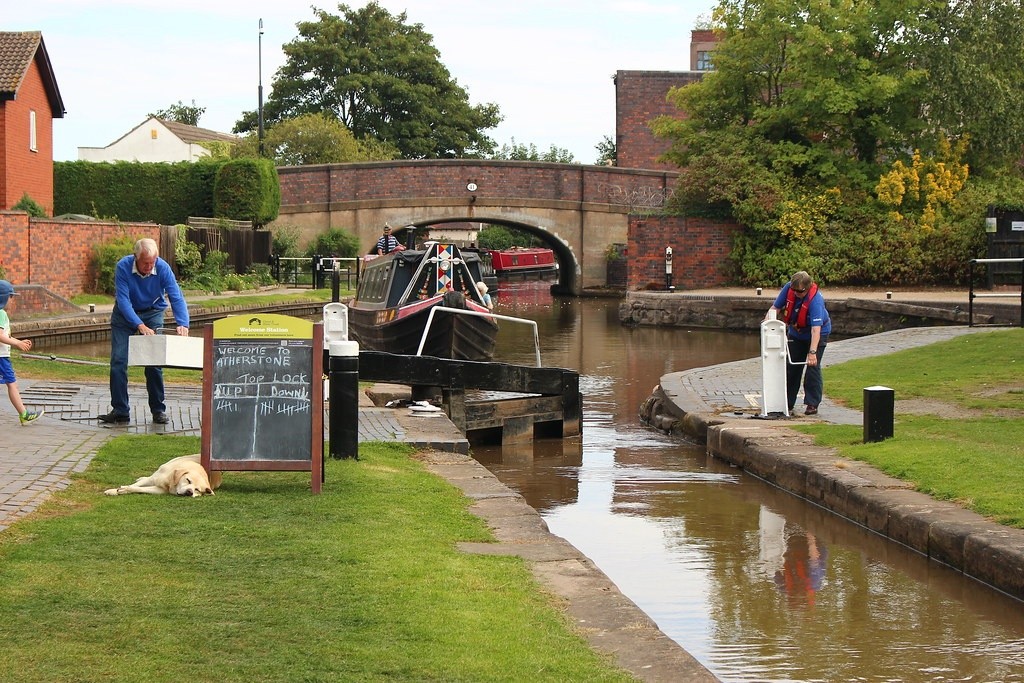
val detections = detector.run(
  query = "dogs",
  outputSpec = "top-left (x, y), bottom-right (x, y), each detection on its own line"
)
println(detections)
top-left (104, 453), bottom-right (222, 498)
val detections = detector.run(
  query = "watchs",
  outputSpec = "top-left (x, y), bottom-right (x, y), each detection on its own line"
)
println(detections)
top-left (809, 349), bottom-right (817, 353)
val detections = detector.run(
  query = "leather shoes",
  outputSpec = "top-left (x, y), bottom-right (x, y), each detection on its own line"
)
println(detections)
top-left (804, 405), bottom-right (818, 415)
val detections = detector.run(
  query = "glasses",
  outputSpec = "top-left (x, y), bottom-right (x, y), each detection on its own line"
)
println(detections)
top-left (791, 288), bottom-right (807, 294)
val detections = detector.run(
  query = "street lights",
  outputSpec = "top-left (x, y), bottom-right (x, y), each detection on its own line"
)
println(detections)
top-left (258, 18), bottom-right (267, 157)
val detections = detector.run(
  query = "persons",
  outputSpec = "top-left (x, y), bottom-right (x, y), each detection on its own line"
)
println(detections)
top-left (98, 238), bottom-right (189, 426)
top-left (475, 282), bottom-right (493, 310)
top-left (0, 280), bottom-right (45, 426)
top-left (760, 271), bottom-right (832, 415)
top-left (377, 225), bottom-right (406, 256)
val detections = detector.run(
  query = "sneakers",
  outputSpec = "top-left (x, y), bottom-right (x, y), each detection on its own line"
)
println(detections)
top-left (20, 410), bottom-right (44, 426)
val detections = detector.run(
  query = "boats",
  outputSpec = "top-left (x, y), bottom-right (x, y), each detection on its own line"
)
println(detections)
top-left (346, 223), bottom-right (501, 363)
top-left (488, 246), bottom-right (559, 278)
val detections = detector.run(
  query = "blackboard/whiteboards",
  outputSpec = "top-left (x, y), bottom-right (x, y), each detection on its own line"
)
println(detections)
top-left (209, 338), bottom-right (314, 462)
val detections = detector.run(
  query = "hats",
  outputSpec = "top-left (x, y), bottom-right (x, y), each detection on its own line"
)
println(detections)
top-left (0, 280), bottom-right (20, 309)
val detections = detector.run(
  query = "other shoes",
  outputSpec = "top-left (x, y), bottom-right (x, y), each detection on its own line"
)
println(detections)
top-left (152, 412), bottom-right (169, 423)
top-left (96, 409), bottom-right (130, 423)
